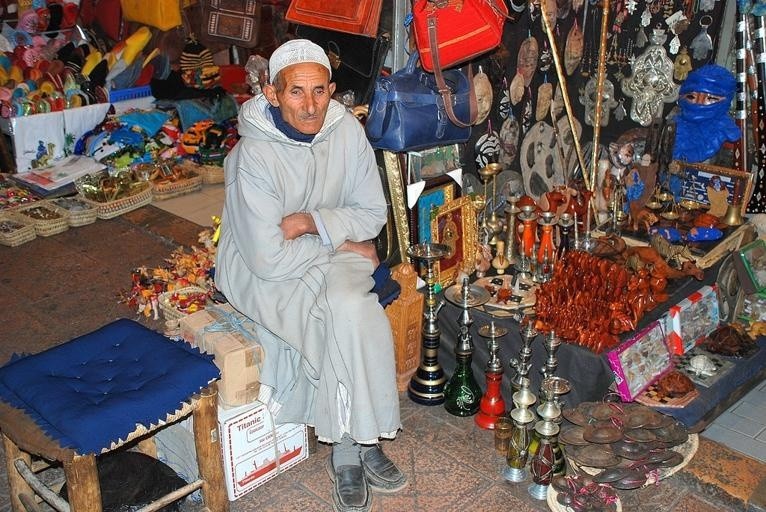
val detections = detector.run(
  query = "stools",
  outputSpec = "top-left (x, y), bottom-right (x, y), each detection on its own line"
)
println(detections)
top-left (1, 317), bottom-right (230, 512)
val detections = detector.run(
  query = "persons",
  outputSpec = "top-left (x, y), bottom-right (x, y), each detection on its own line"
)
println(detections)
top-left (673, 66), bottom-right (743, 163)
top-left (214, 37), bottom-right (407, 511)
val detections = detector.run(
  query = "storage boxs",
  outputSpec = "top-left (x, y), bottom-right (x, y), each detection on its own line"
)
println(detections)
top-left (155, 399), bottom-right (308, 502)
top-left (179, 302), bottom-right (265, 410)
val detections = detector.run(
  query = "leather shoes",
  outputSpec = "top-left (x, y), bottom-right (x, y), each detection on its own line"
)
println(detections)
top-left (325, 447), bottom-right (374, 512)
top-left (362, 444), bottom-right (409, 493)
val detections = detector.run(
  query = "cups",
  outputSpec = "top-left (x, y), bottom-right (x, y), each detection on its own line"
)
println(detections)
top-left (495, 417), bottom-right (514, 457)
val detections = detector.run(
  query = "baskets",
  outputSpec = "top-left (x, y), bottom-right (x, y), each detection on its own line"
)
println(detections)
top-left (0, 151), bottom-right (227, 248)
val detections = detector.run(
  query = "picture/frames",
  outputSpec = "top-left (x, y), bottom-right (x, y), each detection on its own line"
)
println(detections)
top-left (382, 144), bottom-right (460, 266)
top-left (429, 194), bottom-right (476, 294)
top-left (681, 162), bottom-right (754, 217)
top-left (407, 167), bottom-right (463, 274)
